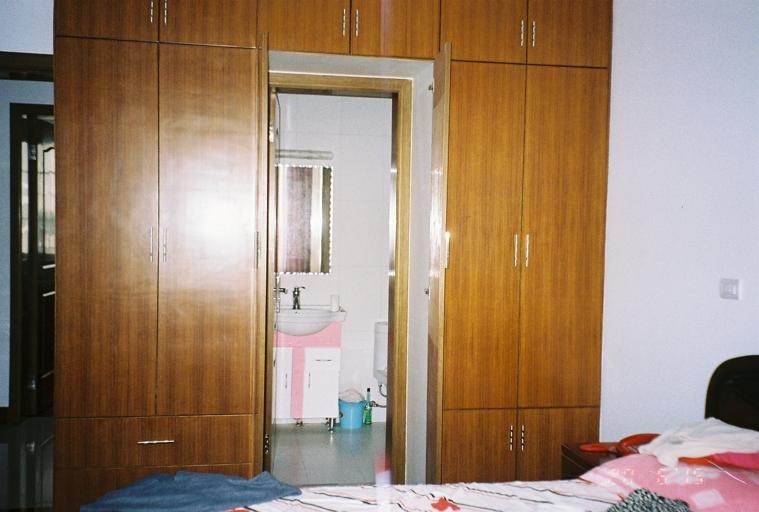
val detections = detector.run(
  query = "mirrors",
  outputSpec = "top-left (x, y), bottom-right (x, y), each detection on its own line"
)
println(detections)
top-left (275, 161), bottom-right (332, 277)
top-left (259, 43), bottom-right (435, 482)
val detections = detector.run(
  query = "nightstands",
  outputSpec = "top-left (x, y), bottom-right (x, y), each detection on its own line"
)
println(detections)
top-left (557, 439), bottom-right (624, 481)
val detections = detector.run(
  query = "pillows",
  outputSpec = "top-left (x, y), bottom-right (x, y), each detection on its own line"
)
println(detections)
top-left (586, 452), bottom-right (758, 511)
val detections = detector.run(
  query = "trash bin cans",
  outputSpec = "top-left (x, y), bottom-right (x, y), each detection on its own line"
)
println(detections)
top-left (338, 399), bottom-right (363, 430)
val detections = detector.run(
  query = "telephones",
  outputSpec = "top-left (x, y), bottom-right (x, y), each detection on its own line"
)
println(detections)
top-left (615, 433), bottom-right (659, 456)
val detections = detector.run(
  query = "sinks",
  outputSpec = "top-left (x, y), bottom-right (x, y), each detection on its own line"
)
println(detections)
top-left (274, 307), bottom-right (333, 337)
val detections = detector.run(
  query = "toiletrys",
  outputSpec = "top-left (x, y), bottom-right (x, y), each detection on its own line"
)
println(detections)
top-left (332, 295), bottom-right (339, 311)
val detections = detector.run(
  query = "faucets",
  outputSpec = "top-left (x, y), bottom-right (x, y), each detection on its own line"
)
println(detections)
top-left (292, 286), bottom-right (306, 308)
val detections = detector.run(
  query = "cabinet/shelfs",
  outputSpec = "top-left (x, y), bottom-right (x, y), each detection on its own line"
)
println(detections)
top-left (267, 322), bottom-right (339, 421)
top-left (51, 0), bottom-right (613, 509)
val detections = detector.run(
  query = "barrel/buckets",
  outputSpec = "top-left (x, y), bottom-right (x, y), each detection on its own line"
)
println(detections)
top-left (339, 390), bottom-right (366, 430)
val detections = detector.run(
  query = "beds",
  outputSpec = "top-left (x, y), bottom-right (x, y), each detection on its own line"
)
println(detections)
top-left (73, 353), bottom-right (758, 511)
